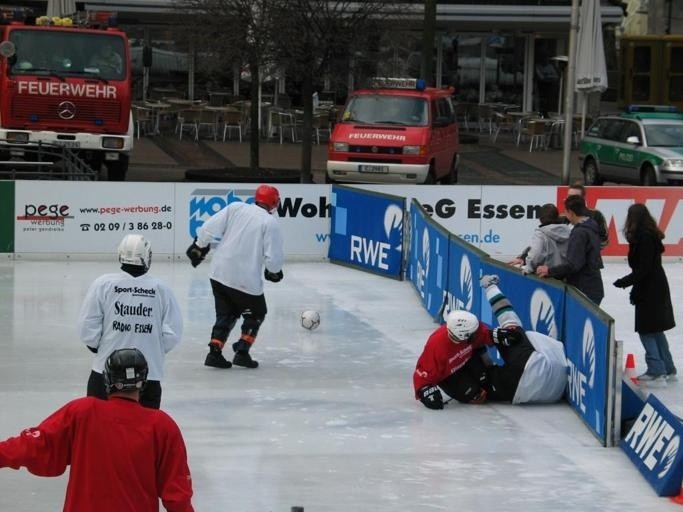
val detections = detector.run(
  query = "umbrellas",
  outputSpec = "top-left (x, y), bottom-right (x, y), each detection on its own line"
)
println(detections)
top-left (572, 0), bottom-right (607, 146)
top-left (46, 0), bottom-right (79, 20)
top-left (238, 5), bottom-right (285, 139)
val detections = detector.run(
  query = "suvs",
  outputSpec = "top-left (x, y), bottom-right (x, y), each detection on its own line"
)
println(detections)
top-left (579, 114), bottom-right (683, 183)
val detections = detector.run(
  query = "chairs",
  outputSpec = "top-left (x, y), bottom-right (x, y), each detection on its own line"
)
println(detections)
top-left (130, 94), bottom-right (251, 143)
top-left (265, 101), bottom-right (341, 145)
top-left (455, 99), bottom-right (587, 155)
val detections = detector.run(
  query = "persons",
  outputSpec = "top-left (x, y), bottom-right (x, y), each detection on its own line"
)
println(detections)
top-left (509, 184), bottom-right (609, 267)
top-left (611, 203), bottom-right (679, 382)
top-left (85, 36), bottom-right (123, 72)
top-left (75, 233), bottom-right (186, 411)
top-left (467, 274), bottom-right (566, 405)
top-left (186, 182), bottom-right (284, 370)
top-left (412, 309), bottom-right (520, 407)
top-left (521, 203), bottom-right (574, 276)
top-left (535, 194), bottom-right (606, 305)
top-left (0, 348), bottom-right (197, 511)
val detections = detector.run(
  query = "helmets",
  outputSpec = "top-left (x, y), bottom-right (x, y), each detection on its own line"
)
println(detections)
top-left (256, 184), bottom-right (280, 210)
top-left (447, 310), bottom-right (479, 342)
top-left (104, 348), bottom-right (147, 396)
top-left (119, 234), bottom-right (152, 269)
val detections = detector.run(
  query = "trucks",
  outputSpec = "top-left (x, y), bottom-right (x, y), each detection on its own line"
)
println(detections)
top-left (0, 19), bottom-right (133, 182)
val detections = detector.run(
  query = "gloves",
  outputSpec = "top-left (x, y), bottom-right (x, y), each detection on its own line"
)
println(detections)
top-left (491, 327), bottom-right (511, 345)
top-left (418, 385), bottom-right (444, 409)
top-left (186, 238), bottom-right (210, 267)
top-left (264, 268), bottom-right (283, 282)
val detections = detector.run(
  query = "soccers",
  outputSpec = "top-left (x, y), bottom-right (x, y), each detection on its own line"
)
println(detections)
top-left (300, 310), bottom-right (321, 330)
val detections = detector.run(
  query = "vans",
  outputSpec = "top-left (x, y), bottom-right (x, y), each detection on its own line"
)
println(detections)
top-left (328, 85), bottom-right (459, 185)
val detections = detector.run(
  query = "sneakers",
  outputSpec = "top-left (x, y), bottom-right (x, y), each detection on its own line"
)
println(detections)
top-left (234, 352), bottom-right (258, 367)
top-left (668, 374), bottom-right (678, 381)
top-left (637, 373), bottom-right (667, 387)
top-left (205, 353), bottom-right (231, 368)
top-left (481, 274), bottom-right (499, 287)
